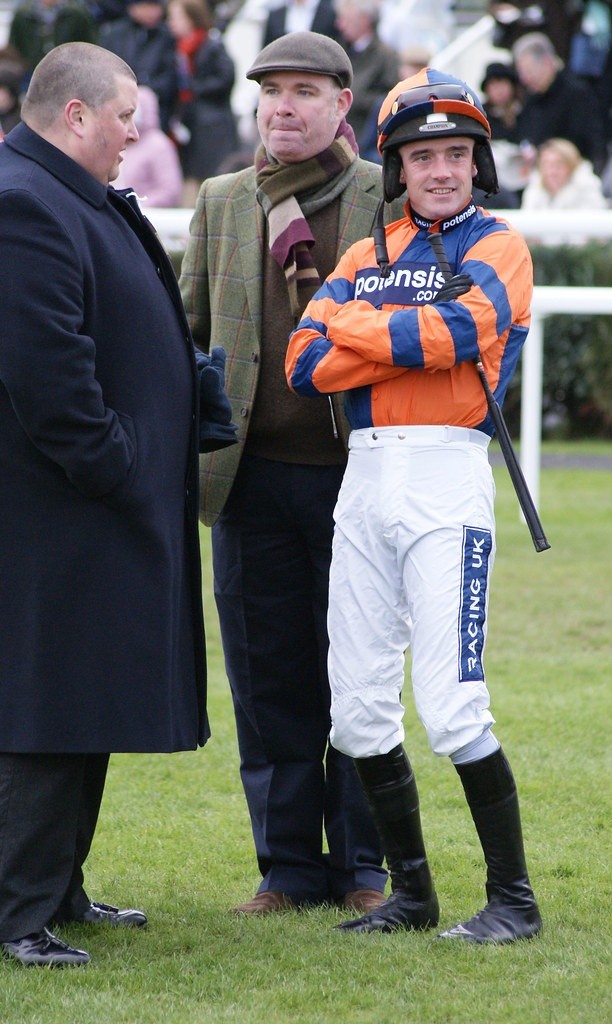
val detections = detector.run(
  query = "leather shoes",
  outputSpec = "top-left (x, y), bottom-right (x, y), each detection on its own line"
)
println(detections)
top-left (226, 890), bottom-right (294, 916)
top-left (55, 902), bottom-right (147, 931)
top-left (0, 925), bottom-right (91, 968)
top-left (343, 888), bottom-right (388, 917)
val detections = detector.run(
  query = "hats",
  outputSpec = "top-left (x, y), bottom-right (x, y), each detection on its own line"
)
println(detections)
top-left (383, 114), bottom-right (501, 205)
top-left (482, 63), bottom-right (517, 94)
top-left (246, 30), bottom-right (354, 91)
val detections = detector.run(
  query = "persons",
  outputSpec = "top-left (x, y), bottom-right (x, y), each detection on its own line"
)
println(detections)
top-left (0, 44), bottom-right (212, 967)
top-left (179, 31), bottom-right (405, 925)
top-left (0, 0), bottom-right (612, 215)
top-left (285, 70), bottom-right (541, 947)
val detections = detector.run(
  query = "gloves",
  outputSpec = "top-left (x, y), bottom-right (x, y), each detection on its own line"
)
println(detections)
top-left (431, 272), bottom-right (474, 303)
top-left (195, 347), bottom-right (238, 454)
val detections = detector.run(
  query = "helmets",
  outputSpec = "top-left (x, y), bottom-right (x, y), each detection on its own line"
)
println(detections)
top-left (377, 66), bottom-right (491, 158)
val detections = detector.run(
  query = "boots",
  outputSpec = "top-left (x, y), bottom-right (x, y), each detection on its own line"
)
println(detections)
top-left (334, 743), bottom-right (441, 934)
top-left (438, 744), bottom-right (543, 947)
